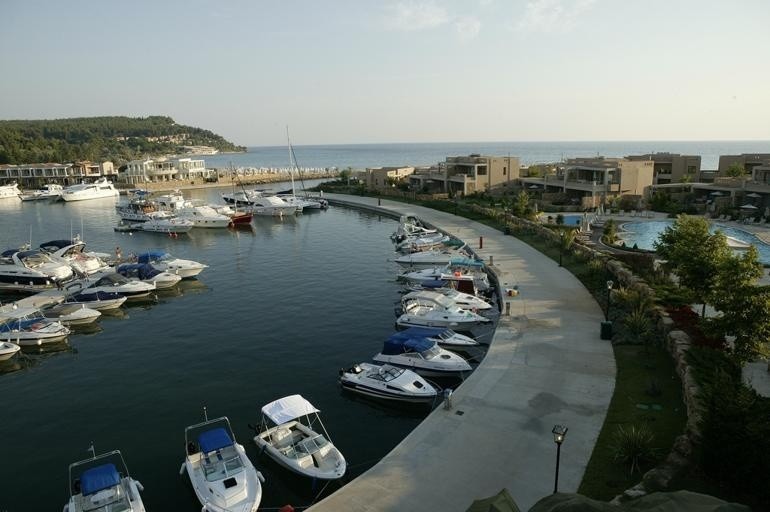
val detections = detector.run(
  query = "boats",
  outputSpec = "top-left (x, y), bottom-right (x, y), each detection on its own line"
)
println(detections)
top-left (333, 358), bottom-right (444, 407)
top-left (257, 215), bottom-right (302, 240)
top-left (0, 197), bottom-right (23, 209)
top-left (16, 182), bottom-right (63, 202)
top-left (232, 223), bottom-right (257, 235)
top-left (178, 405), bottom-right (268, 512)
top-left (0, 183), bottom-right (22, 198)
top-left (115, 188), bottom-right (232, 235)
top-left (247, 393), bottom-right (349, 481)
top-left (0, 211), bottom-right (215, 363)
top-left (370, 334), bottom-right (473, 377)
top-left (59, 176), bottom-right (121, 201)
top-left (65, 195), bottom-right (121, 215)
top-left (0, 355), bottom-right (48, 378)
top-left (212, 202), bottom-right (253, 225)
top-left (388, 211), bottom-right (495, 327)
top-left (60, 438), bottom-right (148, 512)
top-left (399, 326), bottom-right (482, 352)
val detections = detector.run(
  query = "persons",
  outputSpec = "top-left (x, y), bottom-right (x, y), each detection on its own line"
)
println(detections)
top-left (116, 246), bottom-right (123, 261)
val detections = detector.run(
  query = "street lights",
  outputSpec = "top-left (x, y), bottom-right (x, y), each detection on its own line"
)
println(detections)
top-left (557, 230), bottom-right (565, 267)
top-left (502, 206), bottom-right (509, 229)
top-left (550, 423), bottom-right (568, 494)
top-left (605, 278), bottom-right (614, 322)
top-left (454, 192), bottom-right (458, 215)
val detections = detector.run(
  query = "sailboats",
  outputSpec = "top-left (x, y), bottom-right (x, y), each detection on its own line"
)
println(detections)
top-left (223, 122), bottom-right (329, 216)
